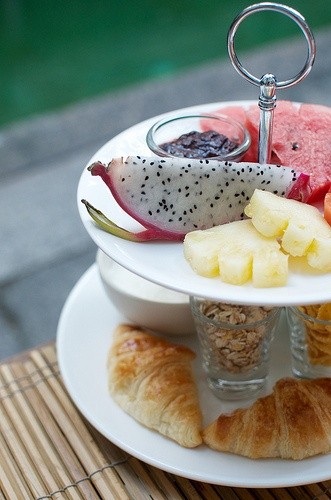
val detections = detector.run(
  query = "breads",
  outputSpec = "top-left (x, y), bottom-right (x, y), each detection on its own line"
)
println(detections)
top-left (105, 322), bottom-right (204, 448)
top-left (202, 376), bottom-right (331, 460)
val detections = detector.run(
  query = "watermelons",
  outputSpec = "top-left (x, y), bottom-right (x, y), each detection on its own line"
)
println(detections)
top-left (197, 101), bottom-right (331, 204)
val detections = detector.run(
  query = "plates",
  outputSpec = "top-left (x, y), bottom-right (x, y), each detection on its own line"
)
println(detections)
top-left (56, 264), bottom-right (331, 488)
top-left (75, 97), bottom-right (331, 308)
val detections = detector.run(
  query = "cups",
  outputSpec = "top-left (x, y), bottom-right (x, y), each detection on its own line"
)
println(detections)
top-left (188, 296), bottom-right (284, 400)
top-left (285, 307), bottom-right (331, 379)
top-left (145, 113), bottom-right (252, 162)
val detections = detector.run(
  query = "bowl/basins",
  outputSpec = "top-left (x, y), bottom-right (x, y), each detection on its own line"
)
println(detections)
top-left (96, 248), bottom-right (198, 337)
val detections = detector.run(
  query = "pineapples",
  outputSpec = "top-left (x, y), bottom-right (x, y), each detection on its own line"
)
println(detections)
top-left (183, 218), bottom-right (288, 287)
top-left (244, 189), bottom-right (331, 273)
